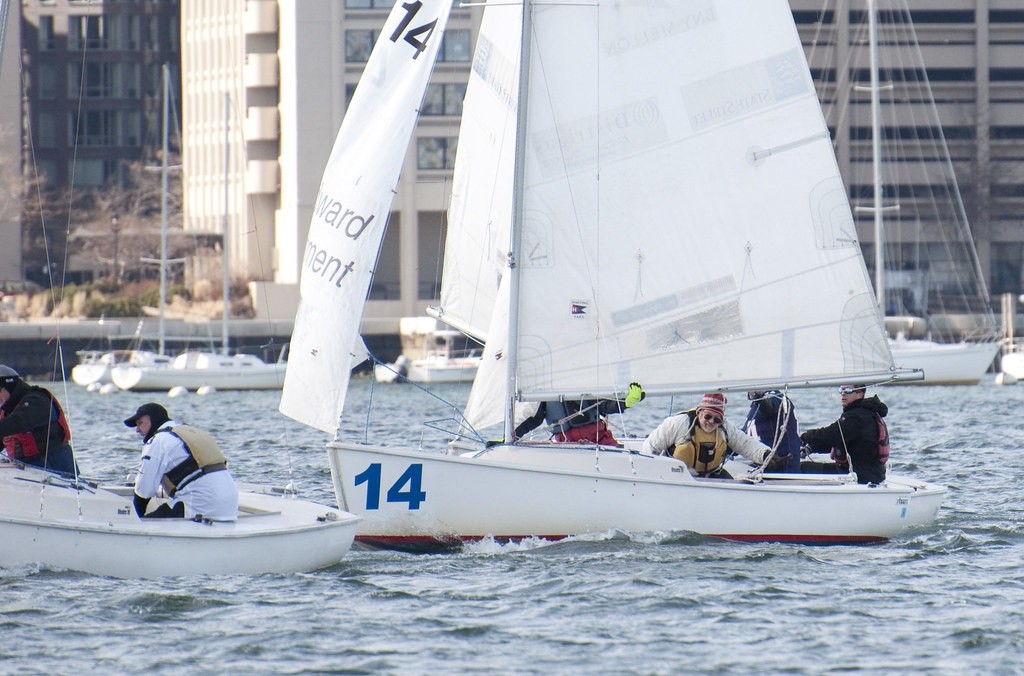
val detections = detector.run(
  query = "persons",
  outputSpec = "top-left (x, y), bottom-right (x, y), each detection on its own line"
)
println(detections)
top-left (122, 402), bottom-right (239, 524)
top-left (0, 363), bottom-right (82, 480)
top-left (798, 384), bottom-right (892, 486)
top-left (647, 392), bottom-right (776, 481)
top-left (740, 391), bottom-right (801, 473)
top-left (512, 383), bottom-right (646, 448)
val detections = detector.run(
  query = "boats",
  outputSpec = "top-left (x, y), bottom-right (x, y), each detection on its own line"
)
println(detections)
top-left (372, 312), bottom-right (485, 386)
top-left (0, 452), bottom-right (363, 583)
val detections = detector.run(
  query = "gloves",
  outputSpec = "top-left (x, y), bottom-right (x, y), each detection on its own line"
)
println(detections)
top-left (623, 382), bottom-right (646, 409)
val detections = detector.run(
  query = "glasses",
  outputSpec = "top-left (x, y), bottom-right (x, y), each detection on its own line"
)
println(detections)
top-left (836, 385), bottom-right (858, 395)
top-left (699, 411), bottom-right (723, 426)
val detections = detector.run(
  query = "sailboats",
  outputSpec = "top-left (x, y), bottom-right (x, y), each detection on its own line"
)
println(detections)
top-left (71, 66), bottom-right (185, 386)
top-left (272, 0), bottom-right (950, 558)
top-left (798, 0), bottom-right (996, 384)
top-left (112, 93), bottom-right (297, 396)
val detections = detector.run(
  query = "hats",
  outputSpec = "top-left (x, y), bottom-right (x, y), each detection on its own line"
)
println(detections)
top-left (0, 363), bottom-right (20, 397)
top-left (121, 401), bottom-right (167, 432)
top-left (696, 393), bottom-right (728, 418)
